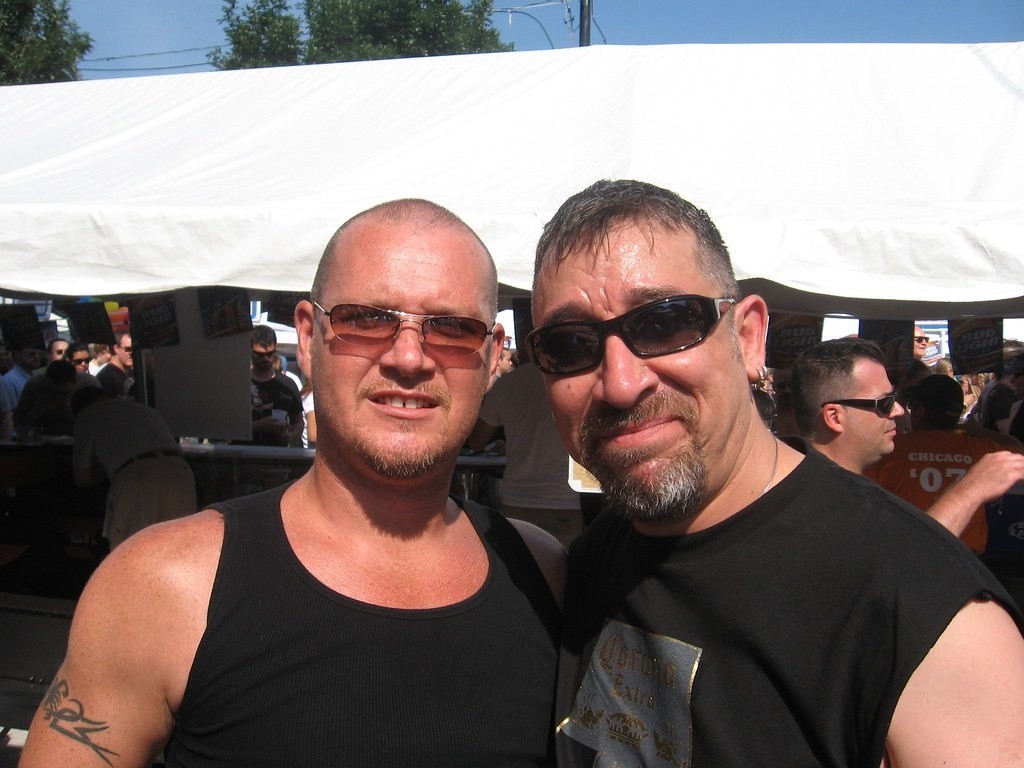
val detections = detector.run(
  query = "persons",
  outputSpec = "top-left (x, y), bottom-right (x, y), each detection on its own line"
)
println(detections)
top-left (531, 176), bottom-right (1024, 768)
top-left (0, 327), bottom-right (317, 551)
top-left (753, 326), bottom-right (1024, 454)
top-left (20, 199), bottom-right (567, 768)
top-left (466, 348), bottom-right (584, 543)
top-left (792, 338), bottom-right (1024, 538)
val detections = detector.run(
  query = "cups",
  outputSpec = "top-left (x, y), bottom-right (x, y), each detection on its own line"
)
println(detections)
top-left (272, 410), bottom-right (287, 418)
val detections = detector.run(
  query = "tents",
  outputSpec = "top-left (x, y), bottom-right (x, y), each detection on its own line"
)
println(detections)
top-left (1, 41), bottom-right (1023, 321)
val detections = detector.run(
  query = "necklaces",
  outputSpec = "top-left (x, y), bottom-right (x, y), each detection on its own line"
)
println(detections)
top-left (761, 428), bottom-right (778, 497)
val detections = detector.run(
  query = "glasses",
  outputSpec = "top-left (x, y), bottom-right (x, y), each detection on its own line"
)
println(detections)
top-left (50, 350), bottom-right (67, 354)
top-left (312, 300), bottom-right (493, 357)
top-left (526, 294), bottom-right (736, 376)
top-left (908, 400), bottom-right (919, 409)
top-left (19, 351), bottom-right (42, 356)
top-left (914, 336), bottom-right (930, 344)
top-left (822, 393), bottom-right (898, 418)
top-left (253, 345), bottom-right (276, 357)
top-left (117, 345), bottom-right (131, 352)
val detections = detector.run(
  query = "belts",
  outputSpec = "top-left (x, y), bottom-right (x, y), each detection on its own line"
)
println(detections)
top-left (114, 450), bottom-right (190, 476)
